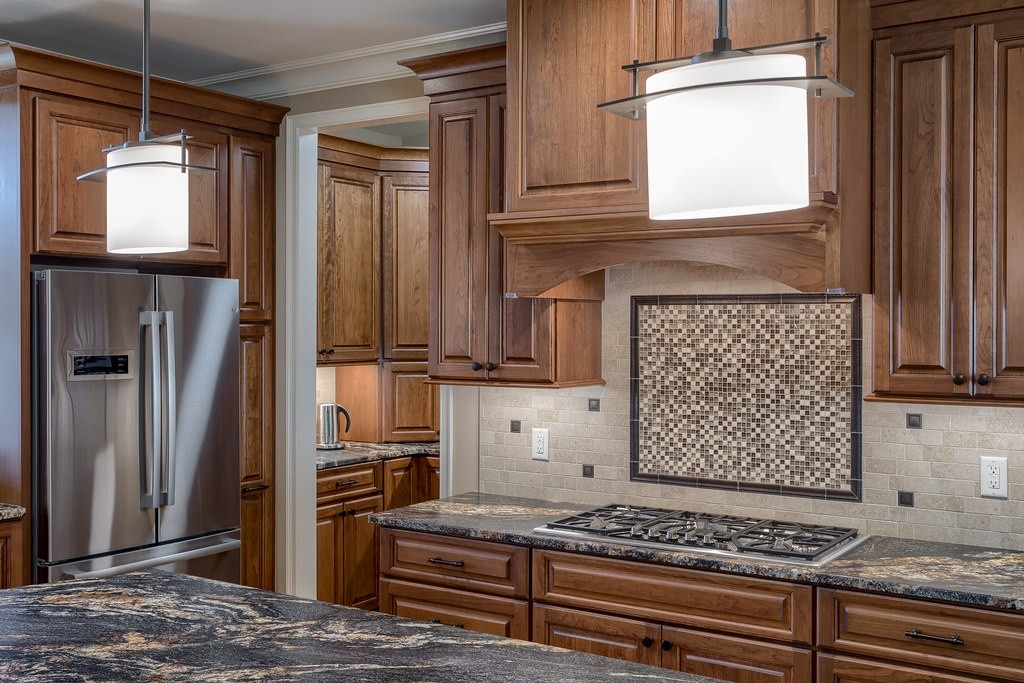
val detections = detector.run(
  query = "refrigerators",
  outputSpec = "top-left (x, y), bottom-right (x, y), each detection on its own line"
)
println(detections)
top-left (30, 262), bottom-right (244, 585)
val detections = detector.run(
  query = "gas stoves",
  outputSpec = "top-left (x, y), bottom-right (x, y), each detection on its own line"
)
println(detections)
top-left (532, 503), bottom-right (870, 570)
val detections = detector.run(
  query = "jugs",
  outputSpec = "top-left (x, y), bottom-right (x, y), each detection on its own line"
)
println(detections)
top-left (316, 403), bottom-right (351, 450)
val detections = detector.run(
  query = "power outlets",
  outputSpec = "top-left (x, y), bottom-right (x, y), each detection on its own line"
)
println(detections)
top-left (531, 428), bottom-right (549, 461)
top-left (980, 457), bottom-right (1008, 498)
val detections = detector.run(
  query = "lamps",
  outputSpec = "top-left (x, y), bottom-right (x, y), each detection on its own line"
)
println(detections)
top-left (75, 0), bottom-right (221, 256)
top-left (597, 0), bottom-right (855, 221)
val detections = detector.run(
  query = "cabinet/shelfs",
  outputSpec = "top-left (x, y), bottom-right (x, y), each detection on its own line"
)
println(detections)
top-left (0, 0), bottom-right (1024, 683)
